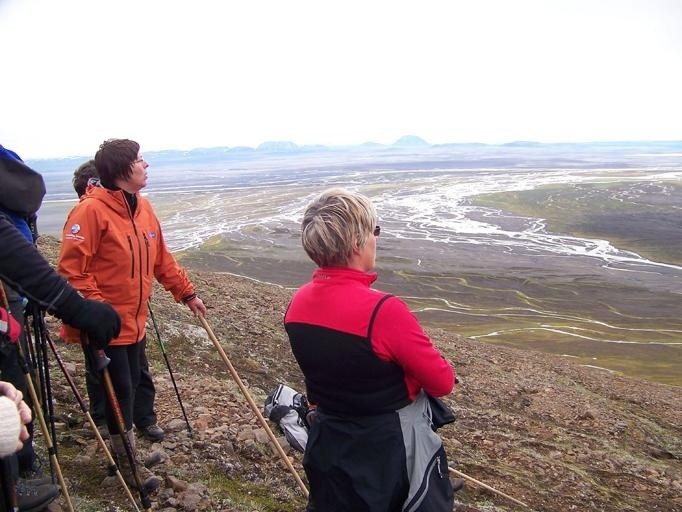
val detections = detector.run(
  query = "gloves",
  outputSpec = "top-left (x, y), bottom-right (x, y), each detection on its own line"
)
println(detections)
top-left (54, 292), bottom-right (121, 351)
top-left (186, 296), bottom-right (206, 318)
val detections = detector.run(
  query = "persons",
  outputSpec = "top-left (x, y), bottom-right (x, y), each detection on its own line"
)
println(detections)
top-left (59, 137), bottom-right (207, 493)
top-left (3, 148), bottom-right (120, 510)
top-left (73, 160), bottom-right (165, 441)
top-left (283, 189), bottom-right (454, 512)
top-left (2, 381), bottom-right (31, 455)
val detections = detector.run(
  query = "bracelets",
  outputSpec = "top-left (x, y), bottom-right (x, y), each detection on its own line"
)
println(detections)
top-left (184, 295), bottom-right (195, 302)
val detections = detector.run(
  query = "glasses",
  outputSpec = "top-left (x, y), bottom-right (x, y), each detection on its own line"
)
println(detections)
top-left (370, 224), bottom-right (380, 237)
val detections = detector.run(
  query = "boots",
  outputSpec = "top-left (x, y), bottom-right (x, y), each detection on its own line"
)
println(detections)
top-left (107, 423), bottom-right (164, 490)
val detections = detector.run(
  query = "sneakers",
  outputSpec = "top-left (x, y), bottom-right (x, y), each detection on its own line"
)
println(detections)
top-left (7, 466), bottom-right (58, 511)
top-left (96, 425), bottom-right (110, 439)
